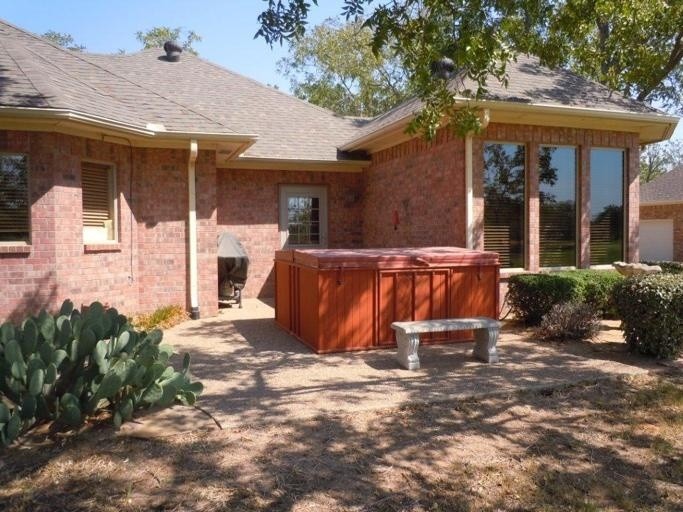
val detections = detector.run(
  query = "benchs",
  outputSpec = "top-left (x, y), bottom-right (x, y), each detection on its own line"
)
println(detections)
top-left (390, 316), bottom-right (502, 370)
